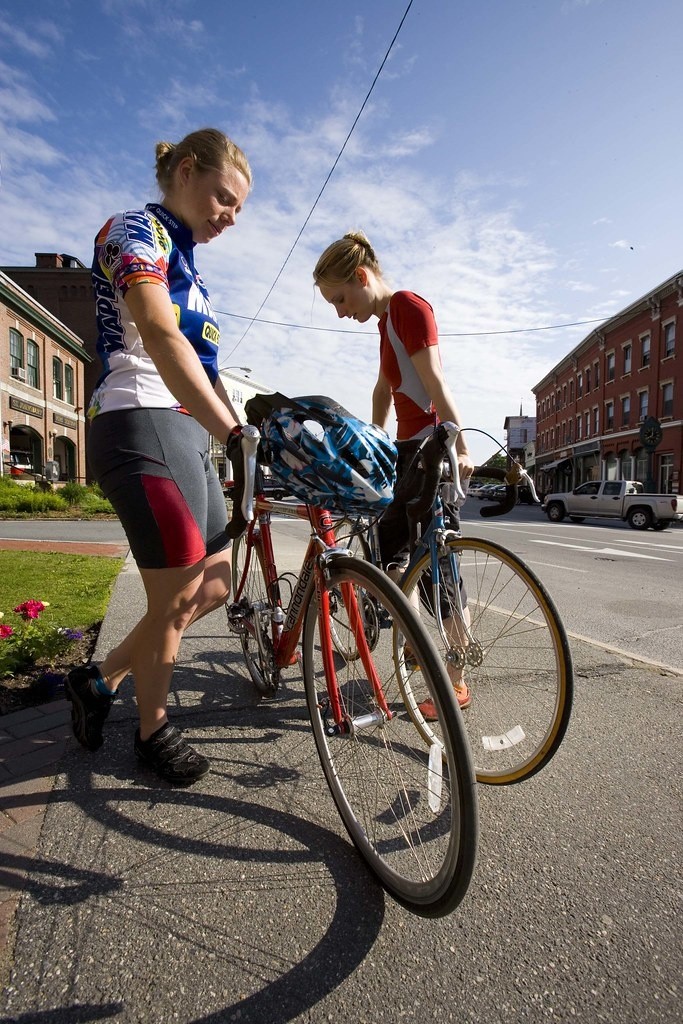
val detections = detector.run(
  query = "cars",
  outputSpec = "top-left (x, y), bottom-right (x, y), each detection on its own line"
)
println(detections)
top-left (466, 480), bottom-right (545, 505)
top-left (227, 478), bottom-right (293, 502)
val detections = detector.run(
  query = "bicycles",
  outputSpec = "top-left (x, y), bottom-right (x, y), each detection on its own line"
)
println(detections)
top-left (203, 421), bottom-right (574, 920)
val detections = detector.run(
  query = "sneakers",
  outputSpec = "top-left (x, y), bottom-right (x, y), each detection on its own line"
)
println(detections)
top-left (417, 684), bottom-right (472, 720)
top-left (134, 721), bottom-right (212, 781)
top-left (392, 644), bottom-right (420, 672)
top-left (63, 665), bottom-right (118, 751)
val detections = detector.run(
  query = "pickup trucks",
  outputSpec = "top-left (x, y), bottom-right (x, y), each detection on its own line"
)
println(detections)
top-left (541, 480), bottom-right (683, 533)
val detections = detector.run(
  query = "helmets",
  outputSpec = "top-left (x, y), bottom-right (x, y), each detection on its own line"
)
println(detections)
top-left (260, 394), bottom-right (398, 515)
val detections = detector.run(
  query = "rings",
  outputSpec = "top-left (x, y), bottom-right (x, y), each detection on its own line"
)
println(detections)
top-left (467, 475), bottom-right (471, 478)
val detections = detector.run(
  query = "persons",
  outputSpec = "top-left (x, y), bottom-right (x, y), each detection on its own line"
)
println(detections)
top-left (313, 231), bottom-right (474, 721)
top-left (63, 128), bottom-right (279, 780)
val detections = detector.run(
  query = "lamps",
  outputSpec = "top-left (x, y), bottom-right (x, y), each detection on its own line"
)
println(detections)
top-left (49, 429), bottom-right (57, 442)
top-left (3, 419), bottom-right (13, 434)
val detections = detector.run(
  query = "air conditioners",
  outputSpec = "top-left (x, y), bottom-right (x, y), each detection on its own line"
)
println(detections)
top-left (12, 367), bottom-right (27, 381)
